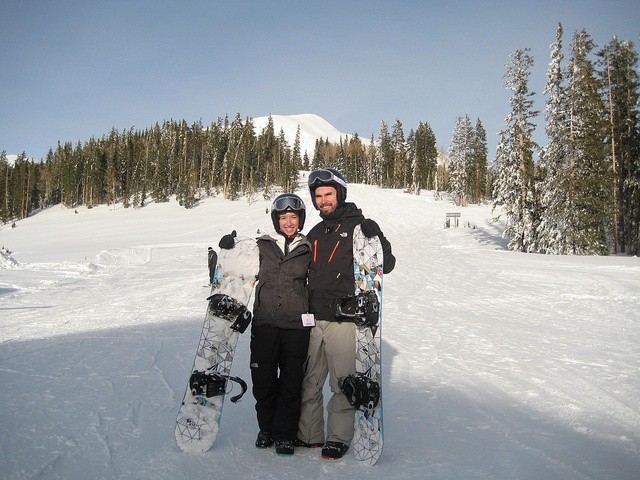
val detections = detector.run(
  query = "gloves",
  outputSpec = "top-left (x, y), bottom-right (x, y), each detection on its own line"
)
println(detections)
top-left (219, 229), bottom-right (237, 248)
top-left (361, 219), bottom-right (384, 239)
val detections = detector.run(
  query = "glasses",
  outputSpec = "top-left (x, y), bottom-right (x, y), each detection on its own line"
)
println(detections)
top-left (274, 198), bottom-right (303, 211)
top-left (308, 170), bottom-right (334, 185)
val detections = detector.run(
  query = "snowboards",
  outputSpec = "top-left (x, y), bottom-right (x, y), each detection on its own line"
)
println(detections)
top-left (175, 235), bottom-right (262, 451)
top-left (331, 223), bottom-right (384, 465)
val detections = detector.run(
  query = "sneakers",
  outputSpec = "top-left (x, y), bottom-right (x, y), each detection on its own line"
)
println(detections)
top-left (275, 436), bottom-right (293, 456)
top-left (255, 429), bottom-right (275, 448)
top-left (321, 441), bottom-right (349, 460)
top-left (294, 436), bottom-right (326, 448)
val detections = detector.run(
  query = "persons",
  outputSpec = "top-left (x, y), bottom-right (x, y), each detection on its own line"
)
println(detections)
top-left (218, 193), bottom-right (312, 457)
top-left (207, 246), bottom-right (218, 284)
top-left (294, 170), bottom-right (396, 459)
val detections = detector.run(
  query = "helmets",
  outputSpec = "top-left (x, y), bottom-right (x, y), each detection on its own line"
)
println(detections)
top-left (271, 194), bottom-right (306, 234)
top-left (309, 169), bottom-right (347, 210)
